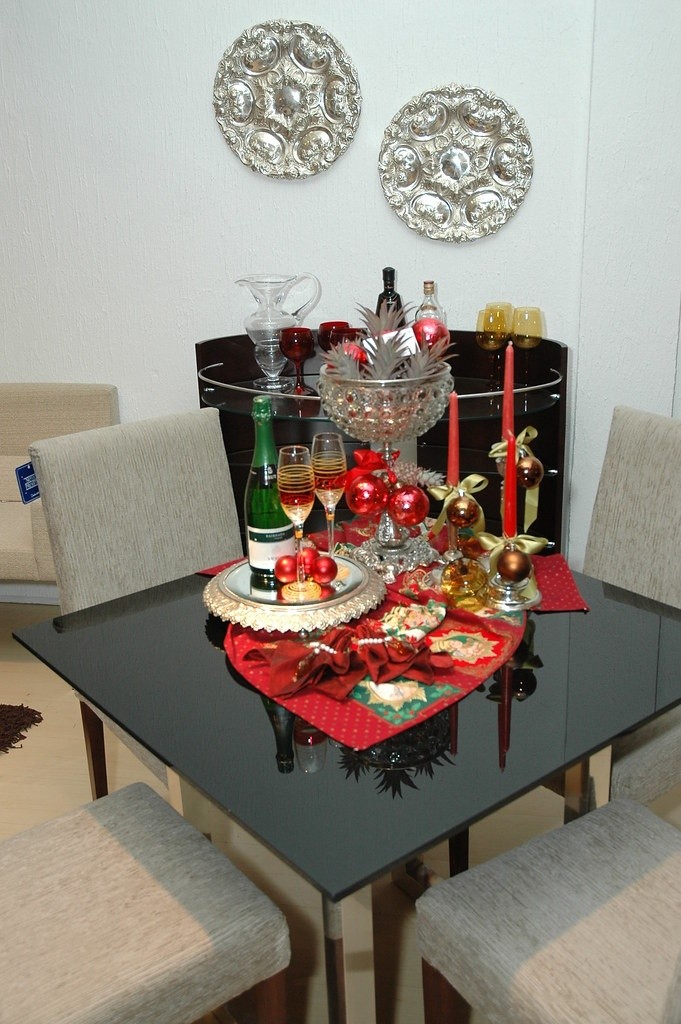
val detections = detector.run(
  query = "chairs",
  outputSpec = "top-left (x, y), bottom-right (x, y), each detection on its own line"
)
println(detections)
top-left (412, 798), bottom-right (681, 1024)
top-left (29, 404), bottom-right (246, 798)
top-left (540, 405), bottom-right (679, 806)
top-left (0, 781), bottom-right (291, 1024)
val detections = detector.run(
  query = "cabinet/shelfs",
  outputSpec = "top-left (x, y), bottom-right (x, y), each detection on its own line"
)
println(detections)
top-left (194, 328), bottom-right (570, 556)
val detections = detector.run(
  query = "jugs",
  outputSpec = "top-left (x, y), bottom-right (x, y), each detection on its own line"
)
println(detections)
top-left (235, 272), bottom-right (322, 394)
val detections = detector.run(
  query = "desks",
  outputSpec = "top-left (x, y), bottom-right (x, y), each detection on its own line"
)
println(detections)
top-left (16, 523), bottom-right (681, 1024)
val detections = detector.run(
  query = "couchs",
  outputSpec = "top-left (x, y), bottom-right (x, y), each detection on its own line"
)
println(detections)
top-left (0, 382), bottom-right (120, 580)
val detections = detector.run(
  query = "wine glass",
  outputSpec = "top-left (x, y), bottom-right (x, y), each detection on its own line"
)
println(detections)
top-left (476, 309), bottom-right (507, 398)
top-left (513, 307), bottom-right (542, 395)
top-left (278, 328), bottom-right (318, 397)
top-left (312, 433), bottom-right (350, 580)
top-left (486, 302), bottom-right (513, 391)
top-left (278, 445), bottom-right (322, 602)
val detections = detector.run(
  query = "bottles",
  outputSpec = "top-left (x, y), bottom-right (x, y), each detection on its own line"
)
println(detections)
top-left (415, 281), bottom-right (445, 325)
top-left (244, 395), bottom-right (295, 600)
top-left (376, 267), bottom-right (406, 328)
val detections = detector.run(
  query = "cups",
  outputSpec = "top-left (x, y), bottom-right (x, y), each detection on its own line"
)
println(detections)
top-left (329, 328), bottom-right (367, 352)
top-left (317, 321), bottom-right (348, 353)
